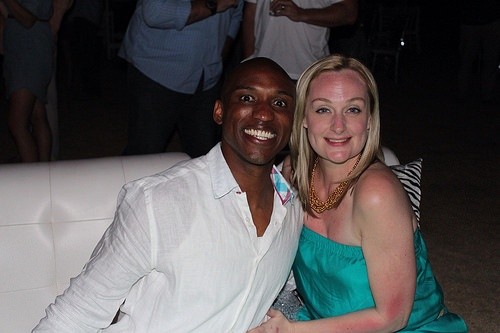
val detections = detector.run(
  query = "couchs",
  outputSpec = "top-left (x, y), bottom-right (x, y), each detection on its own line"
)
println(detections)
top-left (0, 147), bottom-right (400, 333)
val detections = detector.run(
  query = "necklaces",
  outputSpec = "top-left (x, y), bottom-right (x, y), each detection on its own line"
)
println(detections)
top-left (309, 152), bottom-right (364, 214)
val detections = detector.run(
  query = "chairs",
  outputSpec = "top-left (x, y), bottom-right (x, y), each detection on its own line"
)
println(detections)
top-left (352, 0), bottom-right (420, 84)
top-left (97, 8), bottom-right (124, 60)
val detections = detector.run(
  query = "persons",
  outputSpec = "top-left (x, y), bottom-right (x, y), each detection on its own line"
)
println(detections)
top-left (0, 0), bottom-right (356, 164)
top-left (32, 57), bottom-right (303, 333)
top-left (247, 55), bottom-right (470, 333)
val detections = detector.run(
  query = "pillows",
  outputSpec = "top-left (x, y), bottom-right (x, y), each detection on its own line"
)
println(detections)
top-left (388, 158), bottom-right (422, 227)
